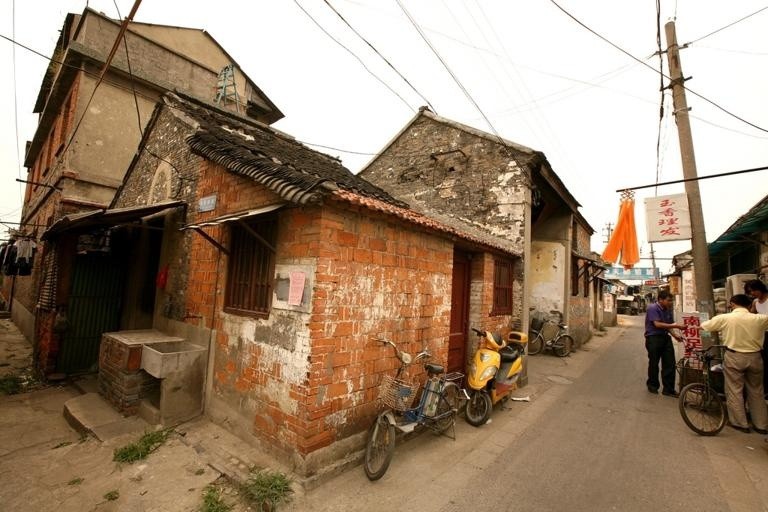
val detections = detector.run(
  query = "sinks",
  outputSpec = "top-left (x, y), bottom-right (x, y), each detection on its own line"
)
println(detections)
top-left (140, 339), bottom-right (207, 379)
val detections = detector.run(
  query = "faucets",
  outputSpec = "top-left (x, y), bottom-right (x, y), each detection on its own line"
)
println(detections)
top-left (181, 310), bottom-right (203, 320)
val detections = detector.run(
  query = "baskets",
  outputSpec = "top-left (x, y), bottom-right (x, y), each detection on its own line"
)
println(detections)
top-left (378, 374), bottom-right (420, 412)
top-left (530, 318), bottom-right (543, 331)
top-left (675, 355), bottom-right (704, 381)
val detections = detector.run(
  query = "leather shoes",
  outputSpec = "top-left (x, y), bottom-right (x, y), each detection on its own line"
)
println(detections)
top-left (661, 390), bottom-right (679, 398)
top-left (753, 423), bottom-right (767, 434)
top-left (648, 388), bottom-right (659, 394)
top-left (727, 422), bottom-right (750, 433)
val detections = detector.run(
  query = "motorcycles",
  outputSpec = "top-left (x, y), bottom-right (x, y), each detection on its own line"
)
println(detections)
top-left (364, 336), bottom-right (464, 481)
top-left (464, 324), bottom-right (529, 425)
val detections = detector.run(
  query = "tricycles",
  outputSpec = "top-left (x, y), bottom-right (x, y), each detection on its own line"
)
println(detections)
top-left (674, 343), bottom-right (747, 436)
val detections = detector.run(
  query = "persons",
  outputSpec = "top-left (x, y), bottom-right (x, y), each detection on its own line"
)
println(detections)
top-left (696, 293), bottom-right (768, 435)
top-left (644, 292), bottom-right (689, 398)
top-left (743, 279), bottom-right (768, 400)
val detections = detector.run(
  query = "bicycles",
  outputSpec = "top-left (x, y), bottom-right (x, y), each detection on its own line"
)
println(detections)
top-left (528, 317), bottom-right (574, 357)
